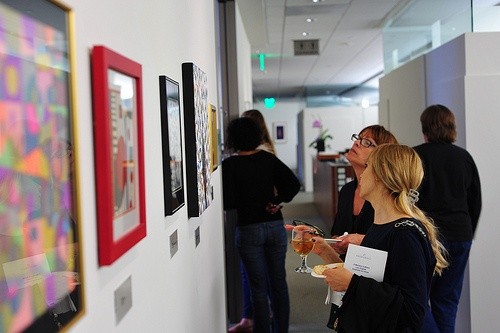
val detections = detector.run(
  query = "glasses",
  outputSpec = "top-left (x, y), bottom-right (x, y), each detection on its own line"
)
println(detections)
top-left (350, 134), bottom-right (377, 148)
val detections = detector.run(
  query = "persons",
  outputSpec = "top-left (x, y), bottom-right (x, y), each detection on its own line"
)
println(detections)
top-left (327, 125), bottom-right (399, 262)
top-left (285, 143), bottom-right (449, 333)
top-left (222, 118), bottom-right (300, 333)
top-left (411, 105), bottom-right (482, 333)
top-left (228, 110), bottom-right (275, 333)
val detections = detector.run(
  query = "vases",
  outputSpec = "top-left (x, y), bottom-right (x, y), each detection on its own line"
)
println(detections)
top-left (317, 138), bottom-right (325, 151)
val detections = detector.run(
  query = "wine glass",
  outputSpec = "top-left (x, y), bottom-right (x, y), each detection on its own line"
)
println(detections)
top-left (292, 225), bottom-right (317, 273)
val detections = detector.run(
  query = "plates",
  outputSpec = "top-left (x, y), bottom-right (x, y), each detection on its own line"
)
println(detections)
top-left (311, 263), bottom-right (346, 278)
top-left (324, 239), bottom-right (341, 243)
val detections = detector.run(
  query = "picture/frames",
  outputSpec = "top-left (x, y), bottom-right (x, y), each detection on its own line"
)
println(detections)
top-left (159, 74), bottom-right (185, 217)
top-left (272, 121), bottom-right (287, 144)
top-left (0, 0), bottom-right (87, 333)
top-left (90, 44), bottom-right (147, 266)
top-left (209, 103), bottom-right (218, 173)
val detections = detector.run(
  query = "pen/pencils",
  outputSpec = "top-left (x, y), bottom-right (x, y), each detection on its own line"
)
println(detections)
top-left (324, 238), bottom-right (342, 242)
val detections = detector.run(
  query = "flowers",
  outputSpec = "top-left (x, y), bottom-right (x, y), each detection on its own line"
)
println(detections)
top-left (308, 119), bottom-right (333, 148)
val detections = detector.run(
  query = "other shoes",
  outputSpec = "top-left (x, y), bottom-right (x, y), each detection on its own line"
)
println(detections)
top-left (228, 322), bottom-right (252, 333)
